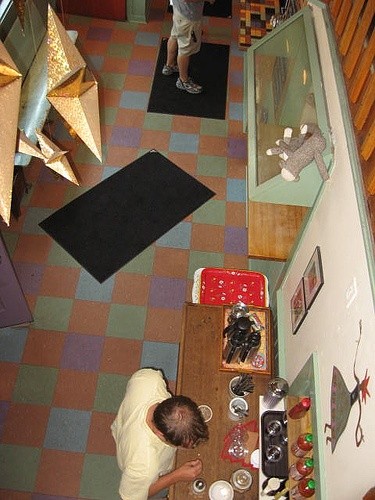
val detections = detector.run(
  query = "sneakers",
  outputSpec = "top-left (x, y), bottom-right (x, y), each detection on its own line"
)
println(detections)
top-left (161, 63), bottom-right (179, 76)
top-left (175, 77), bottom-right (204, 95)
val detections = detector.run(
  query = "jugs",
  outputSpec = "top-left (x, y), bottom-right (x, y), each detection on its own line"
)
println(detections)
top-left (222, 316), bottom-right (262, 364)
top-left (260, 478), bottom-right (290, 500)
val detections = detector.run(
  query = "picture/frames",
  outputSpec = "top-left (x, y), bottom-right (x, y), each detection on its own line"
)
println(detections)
top-left (303, 245), bottom-right (324, 310)
top-left (291, 278), bottom-right (308, 335)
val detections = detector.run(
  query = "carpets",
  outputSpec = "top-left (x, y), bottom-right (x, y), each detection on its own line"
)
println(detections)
top-left (38, 147), bottom-right (216, 285)
top-left (147, 37), bottom-right (230, 120)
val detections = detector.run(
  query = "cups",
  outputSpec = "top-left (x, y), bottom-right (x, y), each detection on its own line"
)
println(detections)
top-left (263, 377), bottom-right (289, 409)
top-left (231, 302), bottom-right (249, 319)
top-left (229, 376), bottom-right (250, 397)
top-left (264, 445), bottom-right (283, 463)
top-left (265, 420), bottom-right (282, 439)
top-left (228, 398), bottom-right (249, 422)
top-left (197, 404), bottom-right (213, 423)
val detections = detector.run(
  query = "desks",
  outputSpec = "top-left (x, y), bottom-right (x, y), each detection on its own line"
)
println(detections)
top-left (168, 300), bottom-right (275, 500)
top-left (192, 267), bottom-right (269, 307)
top-left (13, 29), bottom-right (78, 166)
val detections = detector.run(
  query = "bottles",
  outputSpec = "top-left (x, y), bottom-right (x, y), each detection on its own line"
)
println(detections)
top-left (290, 478), bottom-right (314, 499)
top-left (191, 478), bottom-right (206, 494)
top-left (230, 470), bottom-right (252, 493)
top-left (289, 458), bottom-right (314, 482)
top-left (209, 481), bottom-right (234, 499)
top-left (291, 432), bottom-right (313, 457)
top-left (282, 410), bottom-right (288, 445)
top-left (289, 398), bottom-right (311, 419)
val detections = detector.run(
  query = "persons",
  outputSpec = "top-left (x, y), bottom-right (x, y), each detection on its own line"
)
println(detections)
top-left (162, 0), bottom-right (215, 93)
top-left (111, 368), bottom-right (209, 500)
